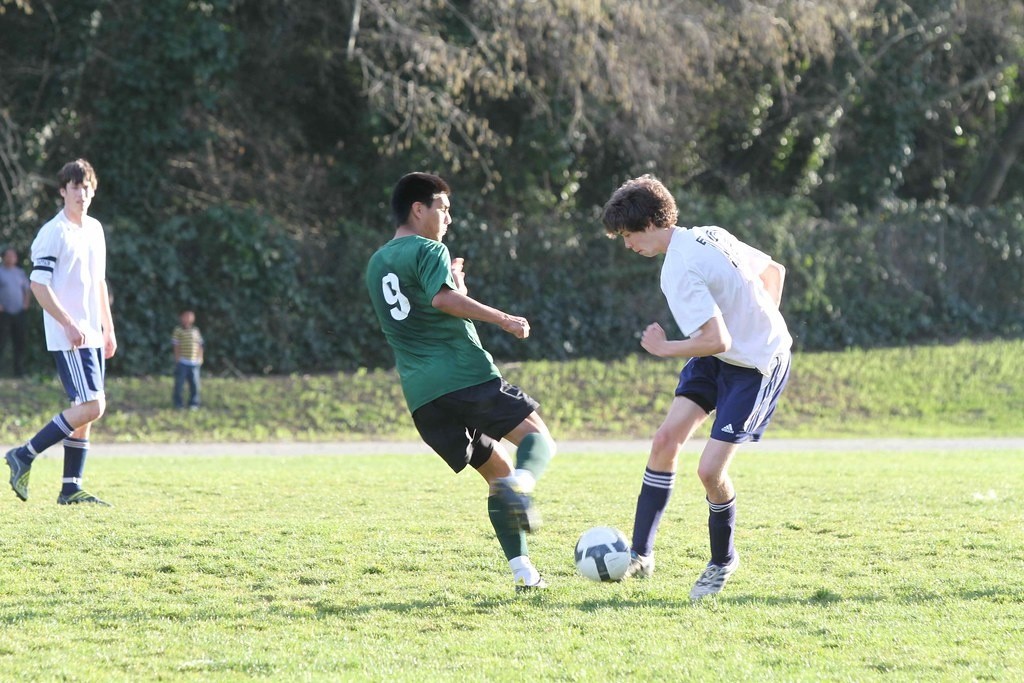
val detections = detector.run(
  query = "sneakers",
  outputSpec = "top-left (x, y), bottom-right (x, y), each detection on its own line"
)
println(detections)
top-left (516, 577), bottom-right (550, 591)
top-left (621, 549), bottom-right (654, 579)
top-left (490, 476), bottom-right (532, 533)
top-left (57, 490), bottom-right (112, 508)
top-left (5, 447), bottom-right (31, 501)
top-left (690, 549), bottom-right (739, 599)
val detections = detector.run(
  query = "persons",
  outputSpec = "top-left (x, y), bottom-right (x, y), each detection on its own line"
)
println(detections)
top-left (602, 172), bottom-right (793, 600)
top-left (367, 172), bottom-right (571, 598)
top-left (4, 158), bottom-right (117, 510)
top-left (0, 248), bottom-right (31, 380)
top-left (171, 308), bottom-right (204, 414)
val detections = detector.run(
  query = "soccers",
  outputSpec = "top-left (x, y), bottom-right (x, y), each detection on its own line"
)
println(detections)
top-left (575, 525), bottom-right (630, 584)
